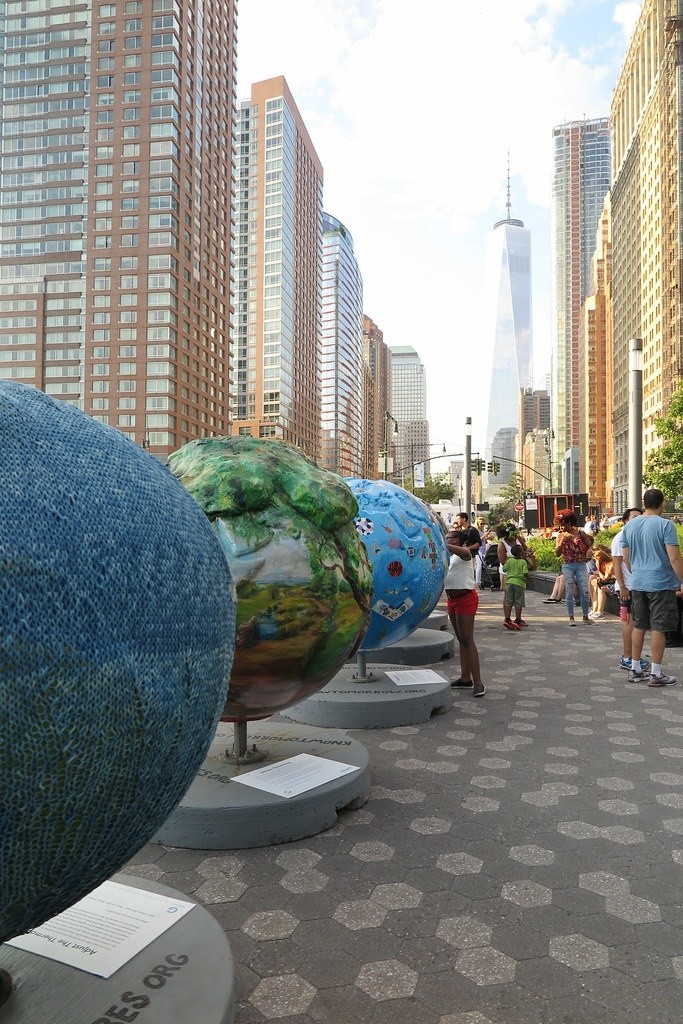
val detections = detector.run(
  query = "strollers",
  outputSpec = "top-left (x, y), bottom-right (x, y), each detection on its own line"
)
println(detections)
top-left (478, 540), bottom-right (501, 592)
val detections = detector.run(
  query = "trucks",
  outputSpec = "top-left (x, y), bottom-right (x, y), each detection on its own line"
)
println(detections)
top-left (524, 492), bottom-right (590, 529)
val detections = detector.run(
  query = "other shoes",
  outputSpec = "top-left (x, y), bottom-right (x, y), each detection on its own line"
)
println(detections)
top-left (473, 683), bottom-right (486, 696)
top-left (544, 599), bottom-right (561, 604)
top-left (450, 678), bottom-right (473, 689)
top-left (542, 599), bottom-right (556, 603)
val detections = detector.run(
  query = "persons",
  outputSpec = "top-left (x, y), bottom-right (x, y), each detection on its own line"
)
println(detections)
top-left (494, 523), bottom-right (529, 632)
top-left (581, 514), bottom-right (611, 536)
top-left (542, 535), bottom-right (617, 618)
top-left (445, 530), bottom-right (486, 696)
top-left (453, 513), bottom-right (493, 590)
top-left (610, 489), bottom-right (683, 687)
top-left (553, 508), bottom-right (598, 626)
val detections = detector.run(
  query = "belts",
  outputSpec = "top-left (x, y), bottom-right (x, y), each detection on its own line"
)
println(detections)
top-left (448, 590), bottom-right (471, 600)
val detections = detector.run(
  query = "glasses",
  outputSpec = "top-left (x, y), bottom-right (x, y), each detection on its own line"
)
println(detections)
top-left (446, 536), bottom-right (454, 539)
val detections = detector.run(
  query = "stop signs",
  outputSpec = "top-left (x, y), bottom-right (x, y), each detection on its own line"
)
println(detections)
top-left (515, 502), bottom-right (525, 511)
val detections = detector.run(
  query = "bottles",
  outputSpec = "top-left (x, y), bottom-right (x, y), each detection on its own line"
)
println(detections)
top-left (620, 601), bottom-right (628, 622)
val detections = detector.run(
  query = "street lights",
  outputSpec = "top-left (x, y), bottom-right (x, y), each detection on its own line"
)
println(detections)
top-left (384, 409), bottom-right (398, 481)
top-left (411, 442), bottom-right (447, 496)
top-left (541, 427), bottom-right (556, 494)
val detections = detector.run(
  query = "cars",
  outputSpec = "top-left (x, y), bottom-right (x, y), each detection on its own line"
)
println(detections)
top-left (609, 516), bottom-right (623, 525)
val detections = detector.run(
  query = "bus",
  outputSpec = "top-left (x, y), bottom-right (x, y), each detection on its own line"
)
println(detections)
top-left (430, 498), bottom-right (476, 528)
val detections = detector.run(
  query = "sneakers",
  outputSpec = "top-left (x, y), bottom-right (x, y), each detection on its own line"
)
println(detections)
top-left (647, 670), bottom-right (678, 686)
top-left (503, 618), bottom-right (521, 630)
top-left (583, 619), bottom-right (599, 626)
top-left (627, 670), bottom-right (649, 682)
top-left (589, 612), bottom-right (605, 620)
top-left (588, 610), bottom-right (596, 616)
top-left (514, 619), bottom-right (528, 626)
top-left (620, 655), bottom-right (649, 671)
top-left (570, 619), bottom-right (576, 625)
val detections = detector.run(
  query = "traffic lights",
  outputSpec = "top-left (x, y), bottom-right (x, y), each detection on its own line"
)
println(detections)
top-left (471, 459), bottom-right (479, 471)
top-left (495, 462), bottom-right (500, 473)
top-left (487, 462), bottom-right (494, 473)
top-left (479, 458), bottom-right (485, 471)
